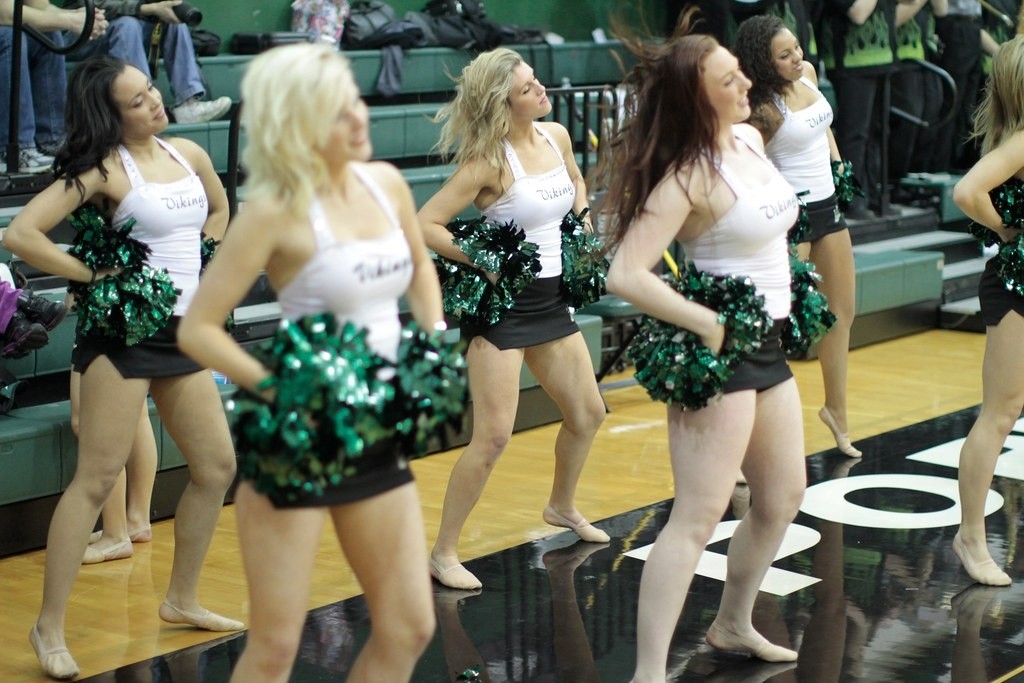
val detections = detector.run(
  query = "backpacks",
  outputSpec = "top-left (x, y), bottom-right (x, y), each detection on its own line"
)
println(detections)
top-left (344, 0), bottom-right (424, 48)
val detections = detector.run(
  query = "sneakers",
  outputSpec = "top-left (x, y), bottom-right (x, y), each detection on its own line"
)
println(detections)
top-left (16, 282), bottom-right (68, 331)
top-left (1, 311), bottom-right (50, 360)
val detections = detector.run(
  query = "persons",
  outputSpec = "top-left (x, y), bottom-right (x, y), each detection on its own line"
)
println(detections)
top-left (597, 6), bottom-right (806, 683)
top-left (0, 0), bottom-right (108, 174)
top-left (63, 0), bottom-right (231, 126)
top-left (733, 14), bottom-right (862, 483)
top-left (416, 48), bottom-right (610, 589)
top-left (4, 56), bottom-right (244, 679)
top-left (178, 45), bottom-right (444, 683)
top-left (952, 38), bottom-right (1024, 585)
top-left (69, 335), bottom-right (158, 563)
top-left (665, 0), bottom-right (1019, 219)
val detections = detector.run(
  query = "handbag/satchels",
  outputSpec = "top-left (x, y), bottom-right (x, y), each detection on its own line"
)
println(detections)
top-left (190, 29), bottom-right (220, 56)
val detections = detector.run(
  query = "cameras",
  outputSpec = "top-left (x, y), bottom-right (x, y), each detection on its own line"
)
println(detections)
top-left (173, 2), bottom-right (202, 27)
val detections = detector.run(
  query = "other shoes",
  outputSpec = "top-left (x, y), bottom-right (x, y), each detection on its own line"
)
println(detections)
top-left (843, 201), bottom-right (877, 220)
top-left (869, 202), bottom-right (902, 217)
top-left (900, 191), bottom-right (940, 207)
top-left (41, 141), bottom-right (65, 155)
top-left (0, 148), bottom-right (55, 174)
top-left (170, 96), bottom-right (232, 125)
